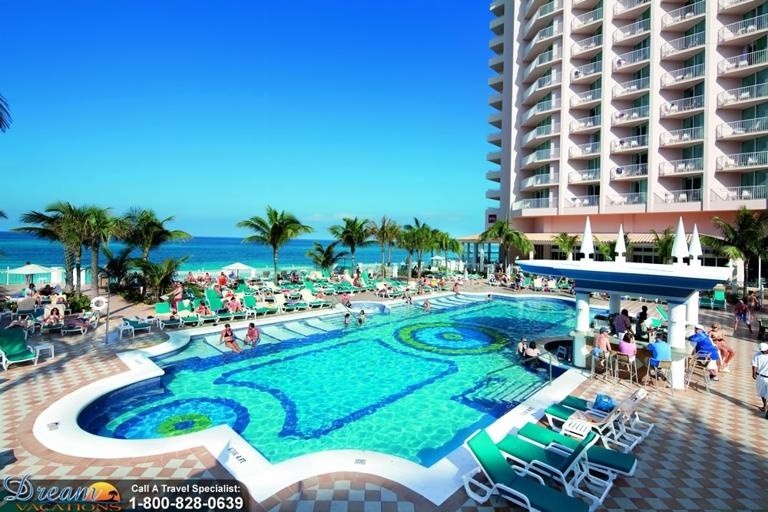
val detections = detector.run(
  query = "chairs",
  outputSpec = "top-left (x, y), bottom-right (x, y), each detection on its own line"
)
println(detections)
top-left (569, 0), bottom-right (759, 209)
top-left (0, 265), bottom-right (730, 512)
top-left (754, 316), bottom-right (768, 339)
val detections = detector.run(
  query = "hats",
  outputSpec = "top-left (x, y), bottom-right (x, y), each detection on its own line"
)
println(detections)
top-left (758, 343), bottom-right (768, 352)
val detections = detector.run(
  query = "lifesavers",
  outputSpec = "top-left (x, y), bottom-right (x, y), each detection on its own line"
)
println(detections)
top-left (91, 297), bottom-right (108, 312)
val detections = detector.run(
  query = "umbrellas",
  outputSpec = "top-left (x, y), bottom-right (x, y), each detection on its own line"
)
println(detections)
top-left (429, 255), bottom-right (445, 267)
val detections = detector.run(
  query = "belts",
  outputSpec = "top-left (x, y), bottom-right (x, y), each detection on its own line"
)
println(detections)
top-left (756, 372), bottom-right (768, 378)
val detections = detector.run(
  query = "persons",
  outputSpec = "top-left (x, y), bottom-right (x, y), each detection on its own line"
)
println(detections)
top-left (6, 262), bottom-right (768, 419)
